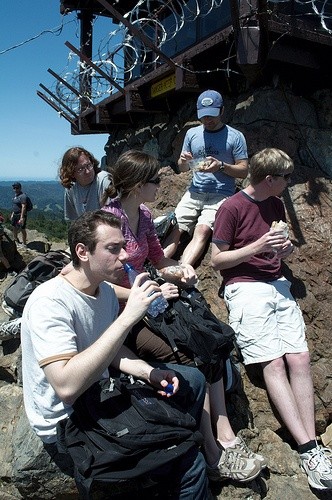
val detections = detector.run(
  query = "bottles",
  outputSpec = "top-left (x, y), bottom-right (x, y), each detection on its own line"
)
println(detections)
top-left (124, 264), bottom-right (169, 318)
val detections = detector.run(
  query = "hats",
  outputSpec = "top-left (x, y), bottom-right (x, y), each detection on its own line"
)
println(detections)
top-left (12, 182), bottom-right (21, 190)
top-left (197, 90), bottom-right (223, 119)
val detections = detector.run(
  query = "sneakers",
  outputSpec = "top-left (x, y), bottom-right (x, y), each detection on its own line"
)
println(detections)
top-left (299, 439), bottom-right (332, 490)
top-left (224, 435), bottom-right (268, 468)
top-left (0, 317), bottom-right (21, 340)
top-left (207, 450), bottom-right (261, 482)
top-left (2, 301), bottom-right (22, 318)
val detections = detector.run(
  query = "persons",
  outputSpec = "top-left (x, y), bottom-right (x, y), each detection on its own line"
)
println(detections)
top-left (10, 183), bottom-right (33, 248)
top-left (57, 147), bottom-right (115, 226)
top-left (61, 150), bottom-right (269, 482)
top-left (157, 89), bottom-right (248, 266)
top-left (210, 147), bottom-right (332, 490)
top-left (19, 209), bottom-right (208, 500)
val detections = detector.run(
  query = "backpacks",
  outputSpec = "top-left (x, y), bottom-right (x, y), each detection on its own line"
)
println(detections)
top-left (155, 213), bottom-right (177, 242)
top-left (143, 257), bottom-right (235, 366)
top-left (57, 375), bottom-right (202, 500)
top-left (18, 193), bottom-right (33, 212)
top-left (3, 250), bottom-right (71, 312)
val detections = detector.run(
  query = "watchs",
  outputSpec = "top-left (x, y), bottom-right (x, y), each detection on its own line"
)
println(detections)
top-left (219, 161), bottom-right (224, 172)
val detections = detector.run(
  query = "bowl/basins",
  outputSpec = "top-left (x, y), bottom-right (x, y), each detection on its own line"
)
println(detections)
top-left (187, 157), bottom-right (214, 172)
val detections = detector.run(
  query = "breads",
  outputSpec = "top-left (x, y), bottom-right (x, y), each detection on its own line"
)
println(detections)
top-left (269, 220), bottom-right (290, 241)
top-left (158, 266), bottom-right (183, 283)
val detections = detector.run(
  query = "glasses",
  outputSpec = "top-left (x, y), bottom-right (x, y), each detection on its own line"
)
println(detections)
top-left (271, 173), bottom-right (292, 181)
top-left (13, 187), bottom-right (19, 189)
top-left (145, 178), bottom-right (161, 184)
top-left (73, 163), bottom-right (95, 172)
